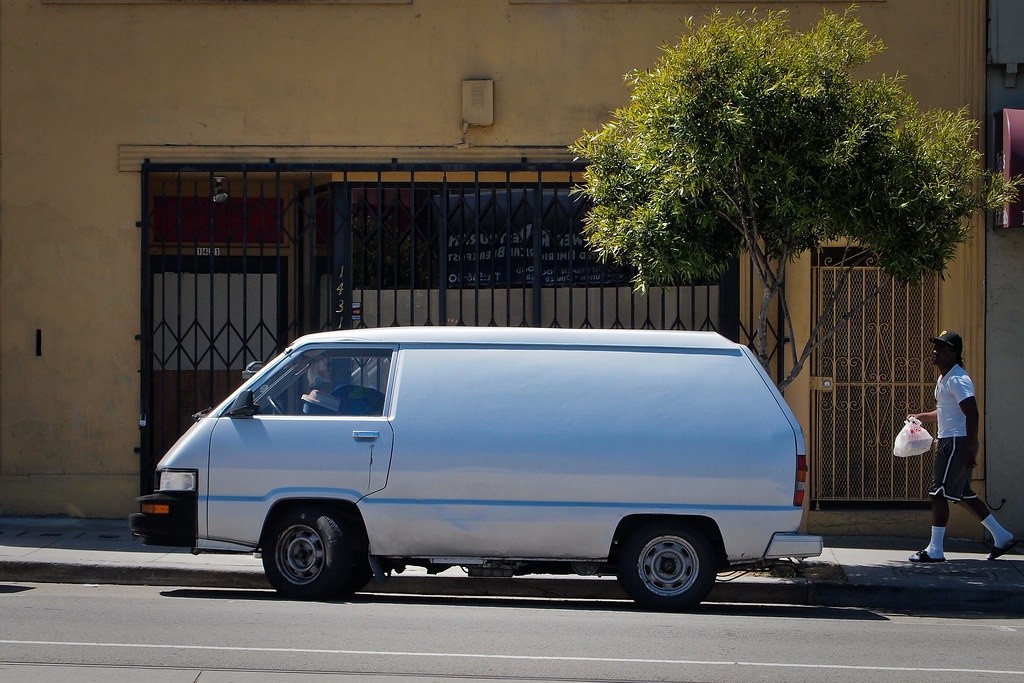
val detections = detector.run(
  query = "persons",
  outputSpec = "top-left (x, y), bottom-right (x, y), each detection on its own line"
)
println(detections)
top-left (906, 331), bottom-right (1017, 563)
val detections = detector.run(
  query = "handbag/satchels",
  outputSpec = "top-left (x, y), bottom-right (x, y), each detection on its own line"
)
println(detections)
top-left (893, 417), bottom-right (933, 457)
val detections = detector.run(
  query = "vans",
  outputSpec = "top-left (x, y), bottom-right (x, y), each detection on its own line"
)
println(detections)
top-left (130, 326), bottom-right (822, 613)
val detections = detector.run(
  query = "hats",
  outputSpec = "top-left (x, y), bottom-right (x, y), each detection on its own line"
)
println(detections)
top-left (929, 331), bottom-right (962, 353)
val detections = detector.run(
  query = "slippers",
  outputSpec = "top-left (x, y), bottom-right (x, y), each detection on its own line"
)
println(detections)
top-left (909, 550), bottom-right (946, 562)
top-left (988, 540), bottom-right (1017, 560)
